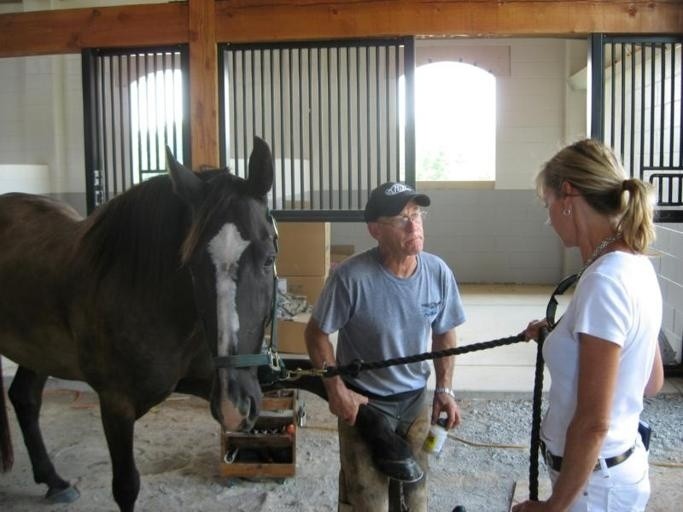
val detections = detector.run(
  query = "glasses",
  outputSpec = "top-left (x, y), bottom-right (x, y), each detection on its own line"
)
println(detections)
top-left (373, 212), bottom-right (427, 227)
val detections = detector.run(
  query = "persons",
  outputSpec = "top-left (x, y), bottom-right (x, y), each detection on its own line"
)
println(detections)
top-left (304, 182), bottom-right (466, 512)
top-left (512, 139), bottom-right (666, 512)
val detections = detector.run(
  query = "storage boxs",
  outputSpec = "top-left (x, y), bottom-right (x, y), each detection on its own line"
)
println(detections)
top-left (269, 279), bottom-right (329, 355)
top-left (273, 221), bottom-right (331, 278)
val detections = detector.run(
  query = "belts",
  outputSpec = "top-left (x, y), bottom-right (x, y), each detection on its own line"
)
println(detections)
top-left (539, 440), bottom-right (631, 471)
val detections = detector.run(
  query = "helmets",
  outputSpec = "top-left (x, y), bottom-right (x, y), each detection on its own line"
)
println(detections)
top-left (365, 182), bottom-right (430, 221)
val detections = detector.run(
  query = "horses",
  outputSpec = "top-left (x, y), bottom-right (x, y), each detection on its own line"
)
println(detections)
top-left (0, 135), bottom-right (425, 512)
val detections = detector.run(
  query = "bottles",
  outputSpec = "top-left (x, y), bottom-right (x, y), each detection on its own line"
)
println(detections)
top-left (421, 418), bottom-right (449, 455)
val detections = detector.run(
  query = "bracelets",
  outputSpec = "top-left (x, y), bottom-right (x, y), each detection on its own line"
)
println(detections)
top-left (435, 388), bottom-right (455, 398)
